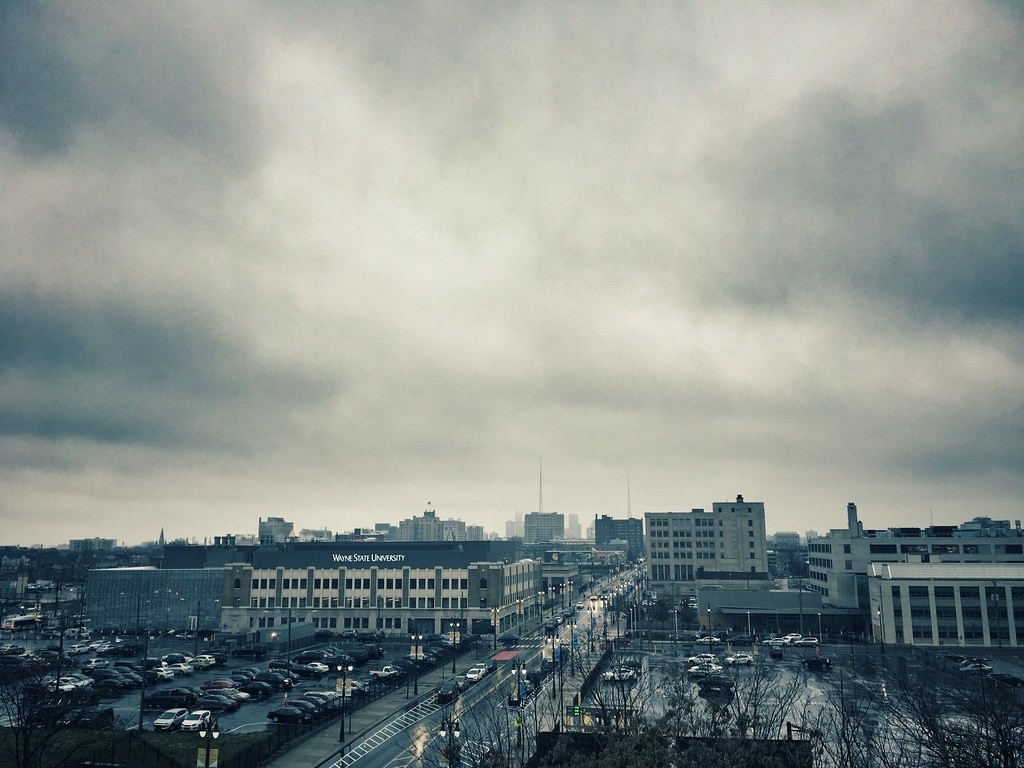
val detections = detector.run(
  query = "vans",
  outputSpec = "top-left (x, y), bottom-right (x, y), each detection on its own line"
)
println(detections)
top-left (78, 707), bottom-right (115, 730)
top-left (56, 706), bottom-right (99, 728)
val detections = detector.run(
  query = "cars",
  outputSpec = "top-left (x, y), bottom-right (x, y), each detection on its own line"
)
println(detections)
top-left (438, 681), bottom-right (460, 701)
top-left (466, 658), bottom-right (498, 683)
top-left (686, 633), bottom-right (831, 691)
top-left (987, 674), bottom-right (1024, 688)
top-left (181, 711), bottom-right (211, 732)
top-left (929, 649), bottom-right (993, 674)
top-left (153, 708), bottom-right (189, 732)
top-left (0, 627), bottom-right (454, 718)
top-left (589, 566), bottom-right (640, 600)
top-left (600, 668), bottom-right (637, 683)
top-left (545, 601), bottom-right (584, 633)
top-left (497, 633), bottom-right (519, 643)
top-left (266, 707), bottom-right (311, 724)
top-left (453, 675), bottom-right (470, 692)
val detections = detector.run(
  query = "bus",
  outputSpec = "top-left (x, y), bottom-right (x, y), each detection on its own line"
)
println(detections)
top-left (3, 616), bottom-right (42, 633)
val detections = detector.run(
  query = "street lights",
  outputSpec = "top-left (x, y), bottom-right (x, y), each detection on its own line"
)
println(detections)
top-left (546, 628), bottom-right (560, 699)
top-left (336, 658), bottom-right (354, 741)
top-left (587, 606), bottom-right (596, 653)
top-left (199, 716), bottom-right (220, 768)
top-left (439, 713), bottom-right (461, 768)
top-left (411, 627), bottom-right (423, 695)
top-left (707, 603), bottom-right (712, 654)
top-left (566, 618), bottom-right (578, 676)
top-left (877, 604), bottom-right (886, 654)
top-left (491, 604), bottom-right (499, 650)
top-left (450, 616), bottom-right (460, 673)
top-left (511, 658), bottom-right (527, 706)
top-left (516, 578), bottom-right (574, 635)
